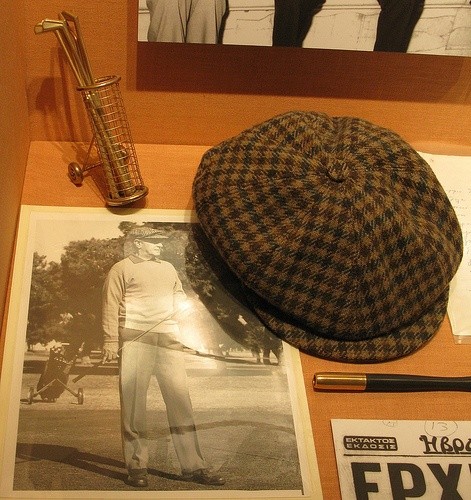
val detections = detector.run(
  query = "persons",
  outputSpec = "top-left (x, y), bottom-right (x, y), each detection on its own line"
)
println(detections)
top-left (101, 227), bottom-right (226, 487)
top-left (272, 1), bottom-right (426, 53)
top-left (145, 0), bottom-right (227, 43)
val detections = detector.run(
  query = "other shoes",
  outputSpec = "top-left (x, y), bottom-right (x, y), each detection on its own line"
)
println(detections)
top-left (184, 470), bottom-right (227, 485)
top-left (127, 471), bottom-right (149, 489)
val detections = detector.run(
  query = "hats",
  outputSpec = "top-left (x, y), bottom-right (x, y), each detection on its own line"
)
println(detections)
top-left (126, 226), bottom-right (169, 241)
top-left (192, 110), bottom-right (464, 364)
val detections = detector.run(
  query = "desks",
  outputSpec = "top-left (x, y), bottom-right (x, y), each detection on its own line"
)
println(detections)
top-left (0, 141), bottom-right (471, 500)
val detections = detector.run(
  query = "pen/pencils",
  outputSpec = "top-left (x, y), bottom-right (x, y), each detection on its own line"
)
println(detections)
top-left (311, 370), bottom-right (469, 395)
